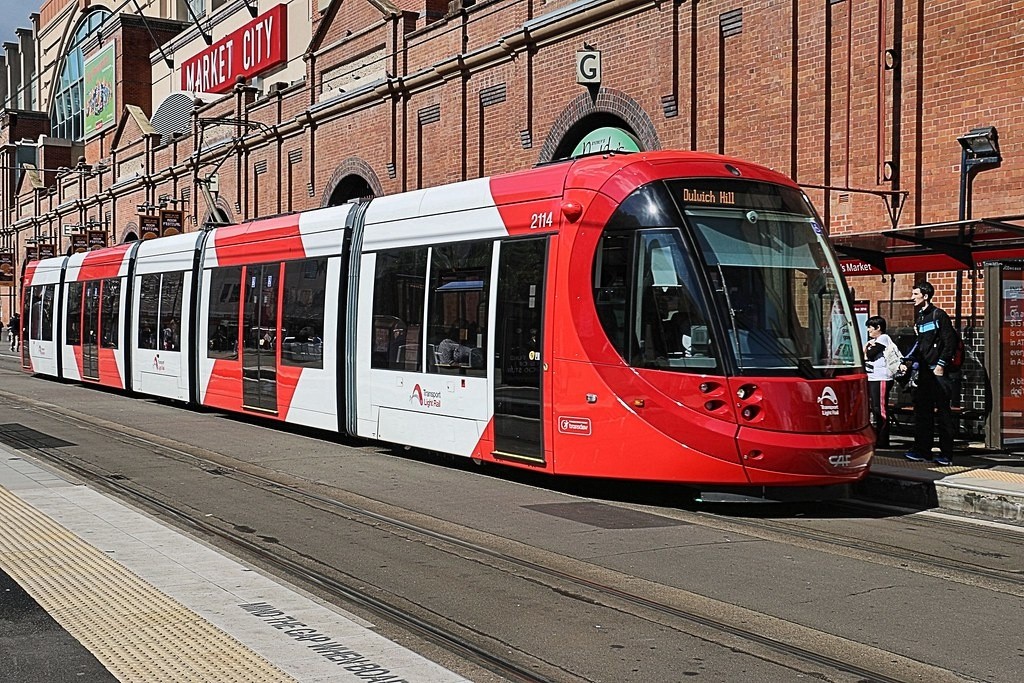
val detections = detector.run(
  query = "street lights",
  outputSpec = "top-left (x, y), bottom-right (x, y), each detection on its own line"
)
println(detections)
top-left (952, 126), bottom-right (1002, 437)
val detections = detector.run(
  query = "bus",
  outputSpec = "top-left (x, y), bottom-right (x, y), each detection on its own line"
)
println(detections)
top-left (19, 126), bottom-right (877, 506)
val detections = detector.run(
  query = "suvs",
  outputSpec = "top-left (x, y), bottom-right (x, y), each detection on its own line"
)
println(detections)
top-left (282, 337), bottom-right (321, 353)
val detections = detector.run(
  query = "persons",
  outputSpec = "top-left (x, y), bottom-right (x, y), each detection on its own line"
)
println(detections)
top-left (742, 277), bottom-right (781, 338)
top-left (900, 282), bottom-right (957, 465)
top-left (865, 316), bottom-right (904, 448)
top-left (10, 313), bottom-right (20, 352)
top-left (0, 317), bottom-right (4, 341)
top-left (438, 319), bottom-right (483, 368)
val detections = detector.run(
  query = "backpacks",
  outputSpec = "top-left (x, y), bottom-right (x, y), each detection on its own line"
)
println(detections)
top-left (933, 308), bottom-right (966, 372)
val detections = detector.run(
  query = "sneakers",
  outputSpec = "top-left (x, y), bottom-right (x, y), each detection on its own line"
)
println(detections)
top-left (905, 450), bottom-right (935, 462)
top-left (934, 457), bottom-right (955, 466)
